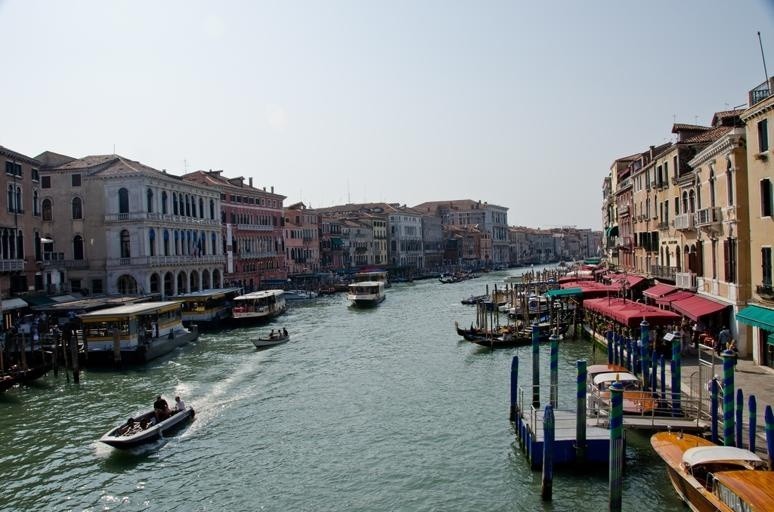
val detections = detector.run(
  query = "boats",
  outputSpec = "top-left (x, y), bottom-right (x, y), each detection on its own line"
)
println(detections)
top-left (98, 406), bottom-right (195, 449)
top-left (251, 329), bottom-right (290, 348)
top-left (587, 364), bottom-right (661, 416)
top-left (650, 431), bottom-right (774, 512)
top-left (439, 273), bottom-right (482, 284)
top-left (233, 289), bottom-right (287, 322)
top-left (455, 293), bottom-right (577, 345)
top-left (346, 281), bottom-right (385, 308)
top-left (285, 287), bottom-right (317, 300)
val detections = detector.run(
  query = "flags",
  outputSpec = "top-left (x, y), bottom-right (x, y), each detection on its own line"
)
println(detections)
top-left (194, 240), bottom-right (203, 259)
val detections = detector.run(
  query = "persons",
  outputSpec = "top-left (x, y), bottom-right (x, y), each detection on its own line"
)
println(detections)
top-left (125, 417), bottom-right (137, 434)
top-left (584, 303), bottom-right (741, 372)
top-left (113, 323), bottom-right (124, 331)
top-left (33, 310), bottom-right (87, 340)
top-left (140, 322), bottom-right (148, 342)
top-left (708, 373), bottom-right (724, 416)
top-left (175, 396), bottom-right (187, 411)
top-left (154, 395), bottom-right (171, 421)
top-left (268, 326), bottom-right (289, 339)
top-left (150, 321), bottom-right (157, 340)
